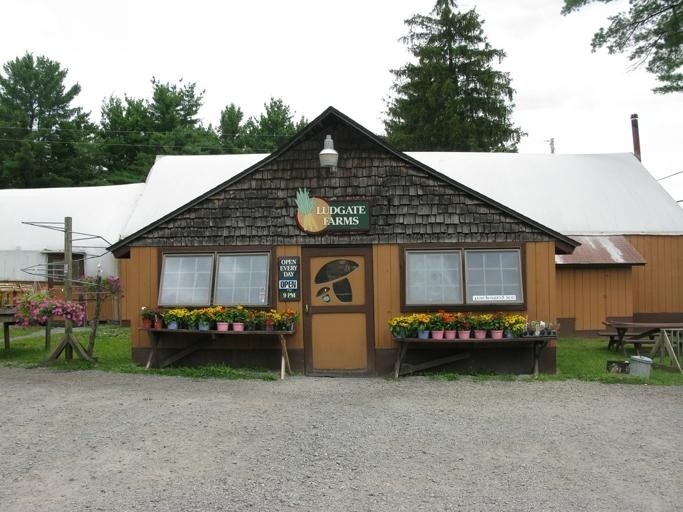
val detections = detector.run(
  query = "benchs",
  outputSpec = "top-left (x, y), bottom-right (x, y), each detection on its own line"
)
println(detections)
top-left (596, 332), bottom-right (676, 359)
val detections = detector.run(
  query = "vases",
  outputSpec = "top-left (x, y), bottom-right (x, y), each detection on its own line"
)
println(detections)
top-left (418, 328), bottom-right (514, 338)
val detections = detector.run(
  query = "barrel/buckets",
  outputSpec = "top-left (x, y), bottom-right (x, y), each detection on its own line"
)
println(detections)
top-left (629, 355), bottom-right (652, 380)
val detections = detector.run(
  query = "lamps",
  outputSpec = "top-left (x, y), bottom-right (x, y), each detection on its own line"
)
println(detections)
top-left (320, 134), bottom-right (339, 174)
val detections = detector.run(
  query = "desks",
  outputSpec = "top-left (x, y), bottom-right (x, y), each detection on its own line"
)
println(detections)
top-left (602, 321), bottom-right (682, 358)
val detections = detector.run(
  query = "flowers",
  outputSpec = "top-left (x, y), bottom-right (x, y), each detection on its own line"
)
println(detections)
top-left (139, 302), bottom-right (300, 331)
top-left (13, 289), bottom-right (85, 328)
top-left (81, 274), bottom-right (121, 293)
top-left (387, 310), bottom-right (528, 337)
